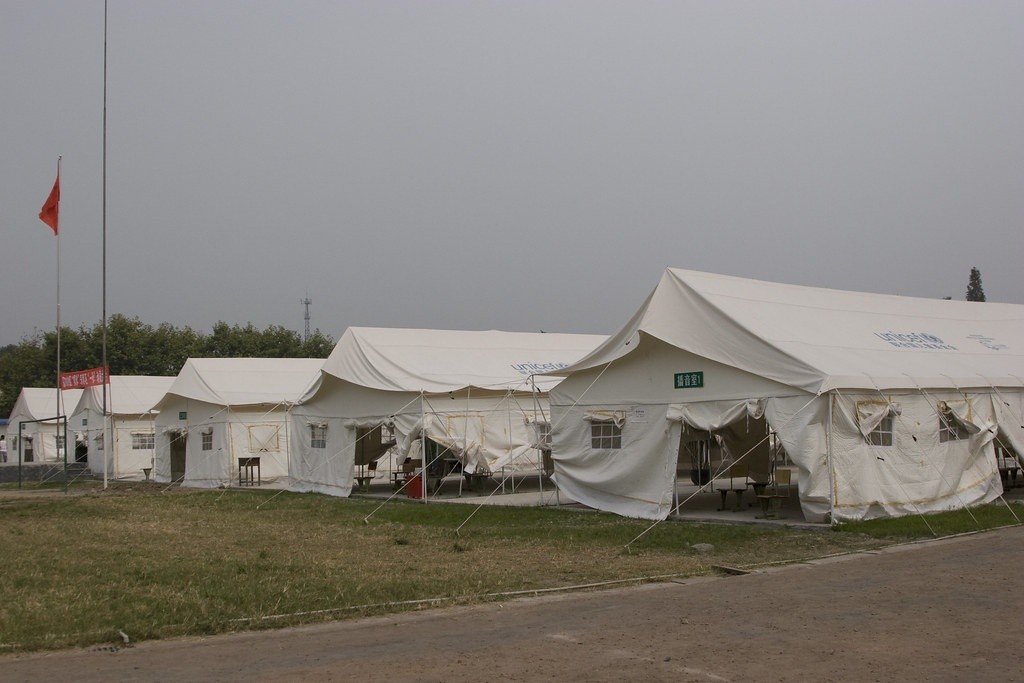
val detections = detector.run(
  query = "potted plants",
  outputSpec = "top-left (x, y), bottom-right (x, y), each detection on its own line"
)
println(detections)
top-left (684, 440), bottom-right (710, 487)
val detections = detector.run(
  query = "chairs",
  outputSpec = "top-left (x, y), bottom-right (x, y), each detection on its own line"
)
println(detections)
top-left (390, 457), bottom-right (492, 497)
top-left (139, 458), bottom-right (154, 482)
top-left (995, 447), bottom-right (1021, 492)
top-left (354, 461), bottom-right (378, 494)
top-left (754, 469), bottom-right (791, 519)
top-left (716, 464), bottom-right (750, 512)
top-left (744, 461), bottom-right (774, 507)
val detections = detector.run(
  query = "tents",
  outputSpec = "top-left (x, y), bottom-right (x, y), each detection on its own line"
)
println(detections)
top-left (5, 266), bottom-right (1024, 528)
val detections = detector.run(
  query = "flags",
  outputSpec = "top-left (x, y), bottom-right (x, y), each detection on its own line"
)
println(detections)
top-left (38, 177), bottom-right (60, 235)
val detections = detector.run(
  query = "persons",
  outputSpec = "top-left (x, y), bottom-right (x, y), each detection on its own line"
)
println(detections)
top-left (0, 434), bottom-right (8, 462)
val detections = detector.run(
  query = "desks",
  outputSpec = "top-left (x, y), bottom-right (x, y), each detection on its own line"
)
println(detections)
top-left (238, 457), bottom-right (261, 487)
